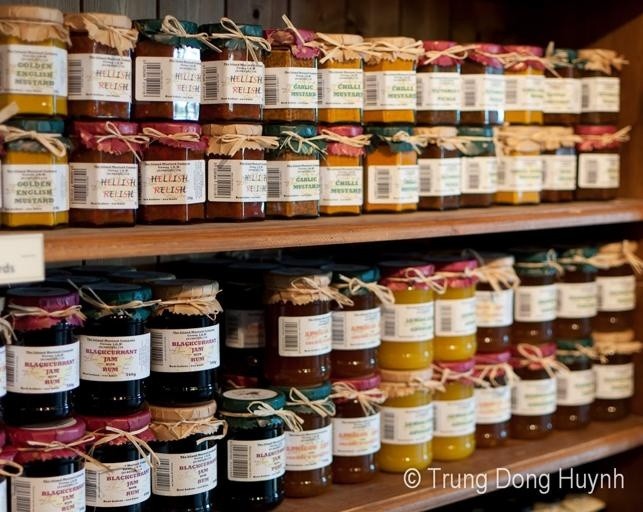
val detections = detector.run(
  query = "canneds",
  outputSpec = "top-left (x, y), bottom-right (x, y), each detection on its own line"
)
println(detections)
top-left (576, 126), bottom-right (622, 201)
top-left (509, 341), bottom-right (558, 441)
top-left (590, 330), bottom-right (635, 423)
top-left (361, 35), bottom-right (418, 125)
top-left (502, 45), bottom-right (543, 125)
top-left (318, 124), bottom-right (364, 215)
top-left (0, 117), bottom-right (72, 228)
top-left (379, 259), bottom-right (434, 369)
top-left (375, 370), bottom-right (434, 474)
top-left (476, 251), bottom-right (514, 352)
top-left (0, 4), bottom-right (70, 118)
top-left (264, 266), bottom-right (333, 389)
top-left (474, 353), bottom-right (511, 448)
top-left (544, 47), bottom-right (581, 126)
top-left (414, 126), bottom-right (459, 211)
top-left (206, 123), bottom-right (267, 222)
top-left (321, 262), bottom-right (382, 380)
top-left (70, 120), bottom-right (139, 228)
top-left (271, 382), bottom-right (332, 499)
top-left (512, 251), bottom-right (557, 345)
top-left (497, 124), bottom-right (543, 205)
top-left (143, 400), bottom-right (221, 511)
top-left (218, 387), bottom-right (285, 509)
top-left (266, 124), bottom-right (320, 220)
top-left (579, 48), bottom-right (619, 125)
top-left (554, 339), bottom-right (595, 430)
top-left (433, 257), bottom-right (478, 362)
top-left (364, 125), bottom-right (419, 212)
top-left (140, 122), bottom-right (206, 224)
top-left (71, 405), bottom-right (156, 512)
top-left (541, 128), bottom-right (579, 203)
top-left (458, 124), bottom-right (495, 209)
top-left (221, 277), bottom-right (265, 367)
top-left (131, 14), bottom-right (202, 124)
top-left (431, 358), bottom-right (477, 462)
top-left (1, 263), bottom-right (223, 427)
top-left (415, 41), bottom-right (461, 125)
top-left (329, 372), bottom-right (381, 486)
top-left (226, 261), bottom-right (280, 284)
top-left (262, 27), bottom-right (319, 123)
top-left (555, 246), bottom-right (598, 341)
top-left (460, 42), bottom-right (502, 126)
top-left (319, 31), bottom-right (364, 124)
top-left (591, 240), bottom-right (638, 334)
top-left (195, 22), bottom-right (266, 122)
top-left (1, 414), bottom-right (86, 512)
top-left (64, 11), bottom-right (138, 121)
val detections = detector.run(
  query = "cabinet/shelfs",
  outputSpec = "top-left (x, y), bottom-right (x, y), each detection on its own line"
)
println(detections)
top-left (0, 198), bottom-right (642, 512)
